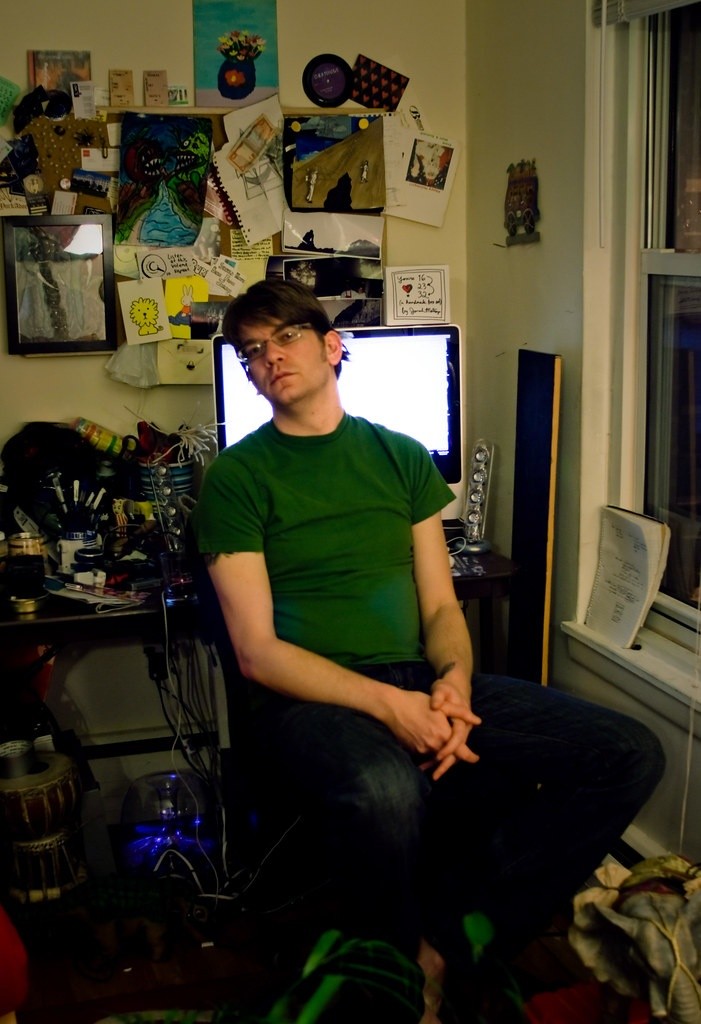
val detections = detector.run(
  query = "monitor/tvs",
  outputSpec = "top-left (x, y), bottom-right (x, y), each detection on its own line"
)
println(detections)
top-left (212, 323), bottom-right (465, 485)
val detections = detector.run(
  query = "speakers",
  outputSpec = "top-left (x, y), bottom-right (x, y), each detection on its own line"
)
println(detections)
top-left (455, 439), bottom-right (493, 555)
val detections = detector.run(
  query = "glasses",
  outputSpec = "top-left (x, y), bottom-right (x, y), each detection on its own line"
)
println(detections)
top-left (236, 322), bottom-right (311, 363)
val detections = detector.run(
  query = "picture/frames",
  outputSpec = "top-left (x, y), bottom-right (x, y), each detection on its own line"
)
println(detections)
top-left (0, 214), bottom-right (117, 354)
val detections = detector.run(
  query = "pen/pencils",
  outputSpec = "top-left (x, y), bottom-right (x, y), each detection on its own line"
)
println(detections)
top-left (52, 475), bottom-right (106, 513)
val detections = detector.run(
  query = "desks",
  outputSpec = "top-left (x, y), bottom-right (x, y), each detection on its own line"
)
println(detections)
top-left (0, 538), bottom-right (522, 760)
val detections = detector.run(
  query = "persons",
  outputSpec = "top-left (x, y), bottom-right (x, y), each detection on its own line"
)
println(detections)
top-left (193, 278), bottom-right (667, 1024)
top-left (301, 229), bottom-right (316, 246)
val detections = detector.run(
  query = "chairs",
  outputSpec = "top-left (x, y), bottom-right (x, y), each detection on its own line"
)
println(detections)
top-left (185, 517), bottom-right (335, 881)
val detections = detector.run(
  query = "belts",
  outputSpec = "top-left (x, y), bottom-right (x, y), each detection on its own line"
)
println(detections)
top-left (357, 661), bottom-right (417, 675)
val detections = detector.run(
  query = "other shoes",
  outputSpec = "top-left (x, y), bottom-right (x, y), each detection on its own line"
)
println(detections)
top-left (430, 943), bottom-right (521, 1024)
